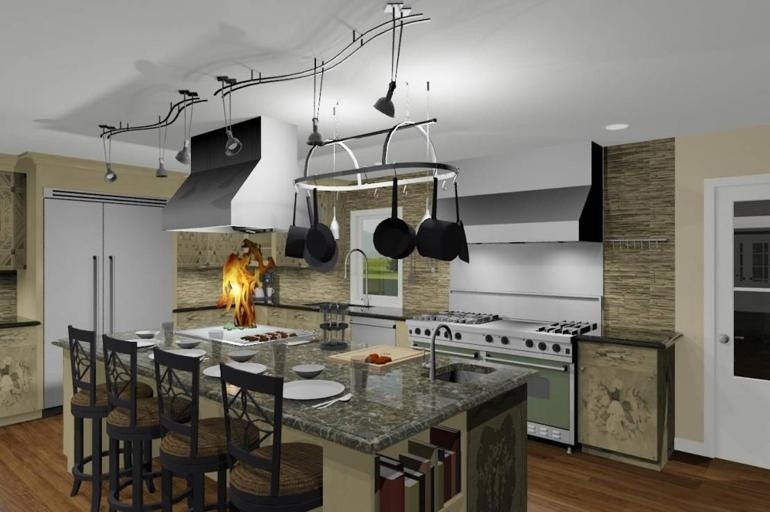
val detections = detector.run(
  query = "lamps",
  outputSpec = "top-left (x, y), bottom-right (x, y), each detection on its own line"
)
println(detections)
top-left (373, 3), bottom-right (411, 118)
top-left (154, 93), bottom-right (199, 179)
top-left (285, 120), bottom-right (469, 268)
top-left (306, 59), bottom-right (329, 147)
top-left (102, 126), bottom-right (117, 182)
top-left (221, 79), bottom-right (244, 158)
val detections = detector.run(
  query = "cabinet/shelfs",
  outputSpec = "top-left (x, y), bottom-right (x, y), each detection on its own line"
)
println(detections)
top-left (0, 171), bottom-right (29, 271)
top-left (576, 341), bottom-right (676, 471)
top-left (0, 327), bottom-right (41, 427)
top-left (174, 306), bottom-right (351, 343)
top-left (177, 230), bottom-right (306, 270)
